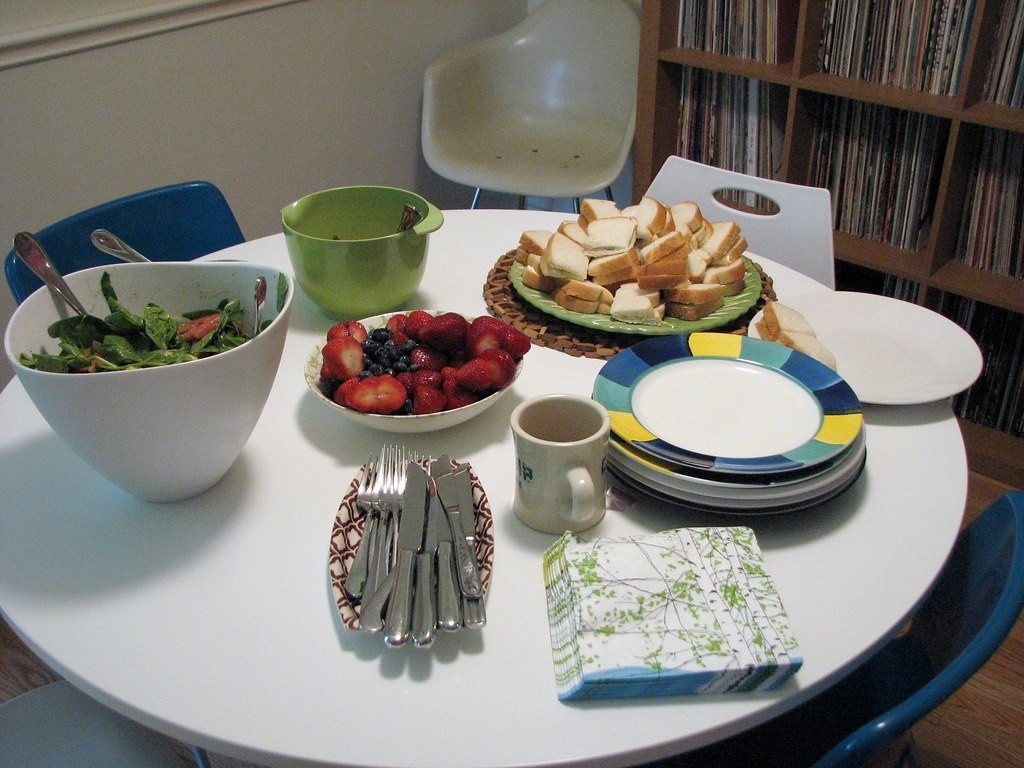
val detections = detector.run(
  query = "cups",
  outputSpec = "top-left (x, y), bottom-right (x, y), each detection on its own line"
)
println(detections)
top-left (511, 396), bottom-right (611, 536)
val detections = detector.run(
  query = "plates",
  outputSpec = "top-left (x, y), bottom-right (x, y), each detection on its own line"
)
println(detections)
top-left (509, 254), bottom-right (763, 335)
top-left (330, 459), bottom-right (494, 631)
top-left (592, 333), bottom-right (867, 517)
top-left (747, 292), bottom-right (983, 405)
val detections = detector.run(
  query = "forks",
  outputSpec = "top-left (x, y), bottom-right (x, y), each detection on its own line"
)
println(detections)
top-left (345, 443), bottom-right (432, 630)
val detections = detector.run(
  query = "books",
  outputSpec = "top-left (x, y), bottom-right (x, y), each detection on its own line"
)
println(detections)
top-left (677, 64), bottom-right (1024, 281)
top-left (835, 259), bottom-right (1024, 440)
top-left (677, 0), bottom-right (1024, 111)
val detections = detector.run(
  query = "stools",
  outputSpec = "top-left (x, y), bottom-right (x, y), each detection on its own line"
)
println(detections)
top-left (0, 685), bottom-right (196, 768)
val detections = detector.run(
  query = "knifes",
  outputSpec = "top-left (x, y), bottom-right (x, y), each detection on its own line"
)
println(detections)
top-left (384, 455), bottom-right (486, 648)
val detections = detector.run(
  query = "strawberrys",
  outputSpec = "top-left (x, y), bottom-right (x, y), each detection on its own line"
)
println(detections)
top-left (320, 310), bottom-right (531, 415)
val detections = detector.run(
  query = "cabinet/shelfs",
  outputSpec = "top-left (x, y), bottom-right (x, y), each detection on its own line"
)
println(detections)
top-left (633, 0), bottom-right (1024, 489)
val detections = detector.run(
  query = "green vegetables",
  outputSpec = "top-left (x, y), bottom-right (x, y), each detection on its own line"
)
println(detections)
top-left (18, 270), bottom-right (289, 374)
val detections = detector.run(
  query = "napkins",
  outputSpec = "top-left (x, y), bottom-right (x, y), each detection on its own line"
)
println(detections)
top-left (540, 526), bottom-right (806, 702)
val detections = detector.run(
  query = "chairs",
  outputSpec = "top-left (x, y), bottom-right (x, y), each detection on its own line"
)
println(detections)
top-left (6, 182), bottom-right (249, 308)
top-left (422, 0), bottom-right (641, 216)
top-left (647, 490), bottom-right (1024, 768)
top-left (643, 154), bottom-right (837, 294)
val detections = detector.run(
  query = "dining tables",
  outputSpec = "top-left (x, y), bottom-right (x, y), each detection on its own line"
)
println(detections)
top-left (0, 210), bottom-right (967, 768)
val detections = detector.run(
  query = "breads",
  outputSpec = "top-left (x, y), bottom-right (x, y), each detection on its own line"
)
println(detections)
top-left (753, 300), bottom-right (837, 374)
top-left (514, 195), bottom-right (748, 325)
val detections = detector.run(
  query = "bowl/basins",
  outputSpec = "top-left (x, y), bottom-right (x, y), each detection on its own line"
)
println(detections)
top-left (281, 185), bottom-right (443, 321)
top-left (304, 310), bottom-right (522, 434)
top-left (3, 261), bottom-right (294, 504)
